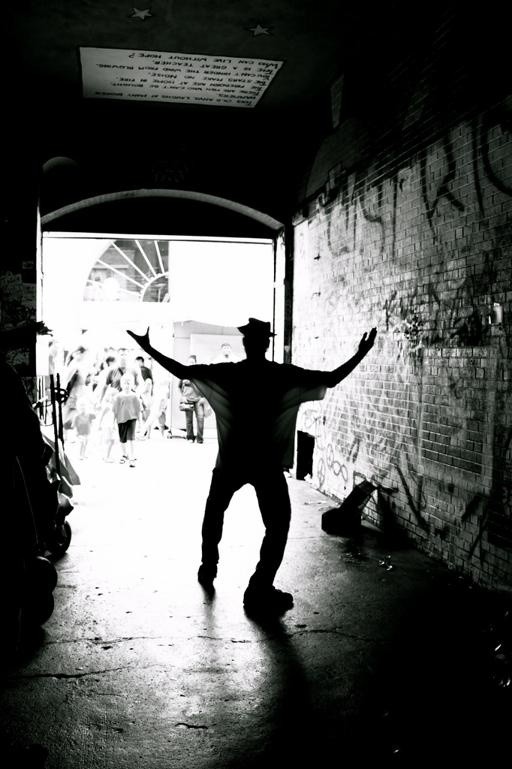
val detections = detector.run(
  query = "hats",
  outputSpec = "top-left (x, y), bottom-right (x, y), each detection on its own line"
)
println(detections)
top-left (238, 318), bottom-right (276, 335)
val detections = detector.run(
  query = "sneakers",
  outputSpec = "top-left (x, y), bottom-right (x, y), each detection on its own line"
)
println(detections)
top-left (120, 454), bottom-right (129, 464)
top-left (199, 563), bottom-right (217, 577)
top-left (243, 587), bottom-right (293, 607)
top-left (130, 458), bottom-right (135, 467)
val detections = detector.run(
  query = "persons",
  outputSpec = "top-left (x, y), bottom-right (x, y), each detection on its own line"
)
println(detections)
top-left (124, 317), bottom-right (379, 610)
top-left (211, 341), bottom-right (234, 364)
top-left (58, 343), bottom-right (208, 464)
